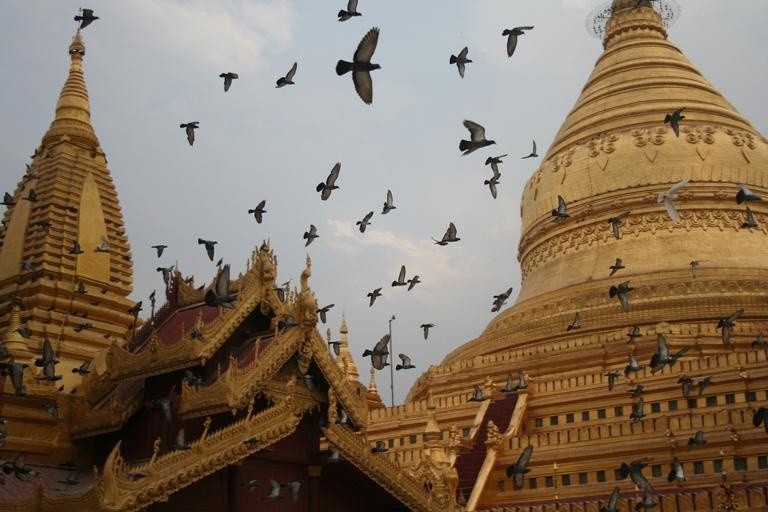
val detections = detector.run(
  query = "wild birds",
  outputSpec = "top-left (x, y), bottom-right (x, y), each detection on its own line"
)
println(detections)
top-left (218, 72), bottom-right (239, 93)
top-left (336, 1), bottom-right (361, 22)
top-left (1, 120), bottom-right (768, 512)
top-left (449, 46), bottom-right (474, 78)
top-left (334, 28), bottom-right (383, 105)
top-left (274, 61), bottom-right (298, 90)
top-left (72, 5), bottom-right (99, 31)
top-left (662, 105), bottom-right (686, 138)
top-left (503, 23), bottom-right (532, 60)
